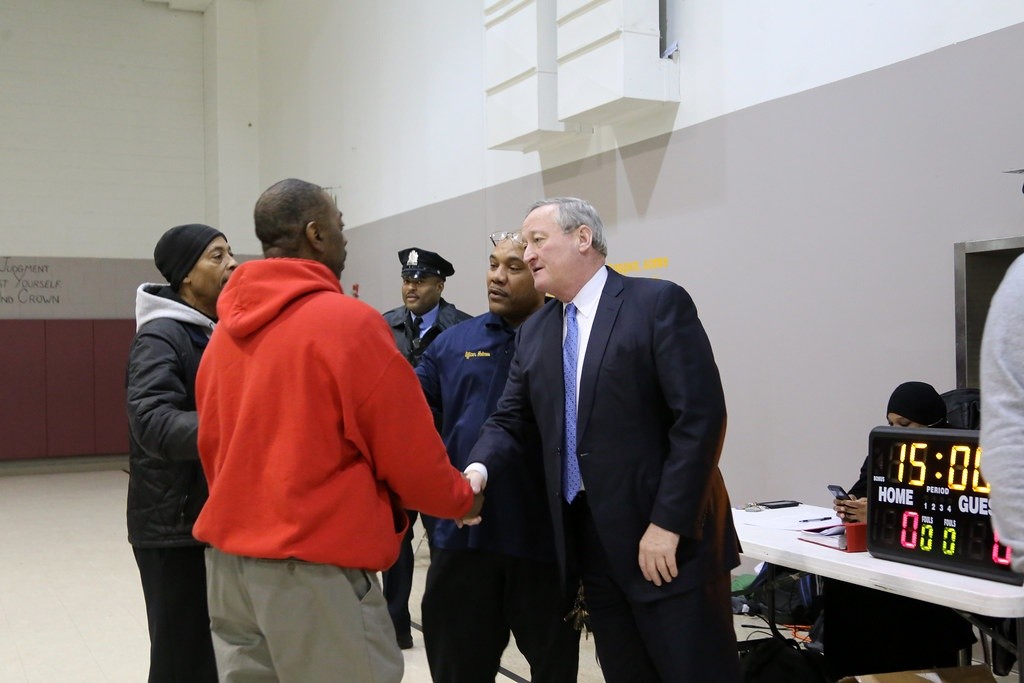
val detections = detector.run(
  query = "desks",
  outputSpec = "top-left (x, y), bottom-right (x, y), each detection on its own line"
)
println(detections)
top-left (732, 503), bottom-right (1024, 683)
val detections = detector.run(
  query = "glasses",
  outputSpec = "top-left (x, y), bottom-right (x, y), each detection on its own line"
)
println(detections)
top-left (489, 230), bottom-right (523, 246)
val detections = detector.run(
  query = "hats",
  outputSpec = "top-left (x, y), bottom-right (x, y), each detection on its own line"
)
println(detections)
top-left (886, 381), bottom-right (948, 428)
top-left (153, 224), bottom-right (230, 292)
top-left (397, 247), bottom-right (455, 280)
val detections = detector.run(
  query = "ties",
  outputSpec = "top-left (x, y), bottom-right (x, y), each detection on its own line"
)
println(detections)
top-left (412, 316), bottom-right (424, 339)
top-left (562, 301), bottom-right (582, 505)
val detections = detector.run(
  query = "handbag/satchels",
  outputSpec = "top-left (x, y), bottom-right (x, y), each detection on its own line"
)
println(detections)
top-left (749, 565), bottom-right (824, 623)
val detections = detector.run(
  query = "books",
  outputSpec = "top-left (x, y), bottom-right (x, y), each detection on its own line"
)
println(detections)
top-left (797, 523), bottom-right (867, 553)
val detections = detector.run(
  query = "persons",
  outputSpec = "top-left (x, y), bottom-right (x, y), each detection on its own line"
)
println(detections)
top-left (978, 254), bottom-right (1024, 575)
top-left (413, 231), bottom-right (580, 683)
top-left (455, 195), bottom-right (744, 683)
top-left (833, 381), bottom-right (951, 524)
top-left (382, 248), bottom-right (474, 649)
top-left (195, 178), bottom-right (484, 683)
top-left (125, 224), bottom-right (237, 683)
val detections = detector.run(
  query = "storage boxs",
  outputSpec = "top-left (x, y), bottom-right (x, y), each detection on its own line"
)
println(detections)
top-left (837, 664), bottom-right (998, 683)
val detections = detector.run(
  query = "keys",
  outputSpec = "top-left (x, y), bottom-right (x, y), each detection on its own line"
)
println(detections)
top-left (563, 586), bottom-right (592, 640)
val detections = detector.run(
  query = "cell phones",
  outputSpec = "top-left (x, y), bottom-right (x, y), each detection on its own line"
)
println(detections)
top-left (827, 485), bottom-right (852, 500)
